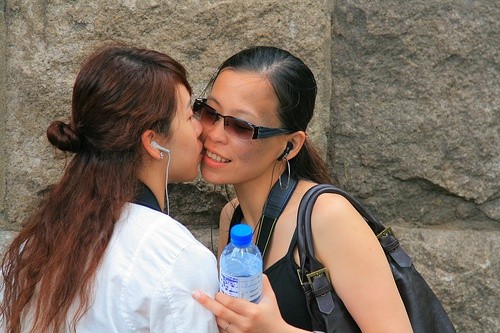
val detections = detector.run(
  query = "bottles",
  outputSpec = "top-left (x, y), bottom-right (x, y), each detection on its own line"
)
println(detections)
top-left (220, 224), bottom-right (263, 305)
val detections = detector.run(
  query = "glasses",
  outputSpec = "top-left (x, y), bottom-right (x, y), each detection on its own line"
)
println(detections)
top-left (193, 98), bottom-right (295, 140)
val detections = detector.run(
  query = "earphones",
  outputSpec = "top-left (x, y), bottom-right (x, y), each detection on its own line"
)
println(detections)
top-left (149, 141), bottom-right (169, 154)
top-left (277, 142), bottom-right (293, 161)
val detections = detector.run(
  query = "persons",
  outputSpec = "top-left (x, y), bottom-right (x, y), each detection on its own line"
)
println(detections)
top-left (0, 37), bottom-right (225, 332)
top-left (191, 44), bottom-right (417, 333)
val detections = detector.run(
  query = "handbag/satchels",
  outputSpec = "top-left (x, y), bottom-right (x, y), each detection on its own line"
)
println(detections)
top-left (295, 185), bottom-right (458, 333)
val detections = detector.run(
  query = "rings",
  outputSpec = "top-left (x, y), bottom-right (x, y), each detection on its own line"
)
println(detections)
top-left (224, 321), bottom-right (229, 331)
